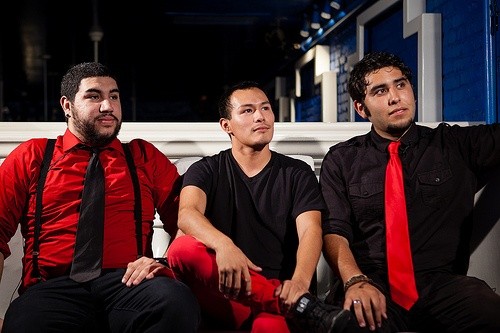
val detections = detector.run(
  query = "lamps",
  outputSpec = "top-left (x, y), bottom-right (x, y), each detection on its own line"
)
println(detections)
top-left (300, 0), bottom-right (340, 38)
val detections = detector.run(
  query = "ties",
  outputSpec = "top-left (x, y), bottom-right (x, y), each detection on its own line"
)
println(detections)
top-left (385, 142), bottom-right (419, 312)
top-left (69, 142), bottom-right (114, 283)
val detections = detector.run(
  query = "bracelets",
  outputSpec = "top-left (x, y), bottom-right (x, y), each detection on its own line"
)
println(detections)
top-left (344, 274), bottom-right (383, 293)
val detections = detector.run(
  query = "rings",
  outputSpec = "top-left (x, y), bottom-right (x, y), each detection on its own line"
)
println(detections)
top-left (353, 300), bottom-right (361, 304)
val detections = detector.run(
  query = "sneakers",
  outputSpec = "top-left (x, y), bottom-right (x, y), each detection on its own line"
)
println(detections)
top-left (296, 294), bottom-right (351, 333)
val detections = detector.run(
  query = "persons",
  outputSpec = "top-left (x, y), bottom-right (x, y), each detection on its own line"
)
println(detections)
top-left (0, 62), bottom-right (202, 333)
top-left (319, 52), bottom-right (500, 333)
top-left (166, 81), bottom-right (352, 333)
